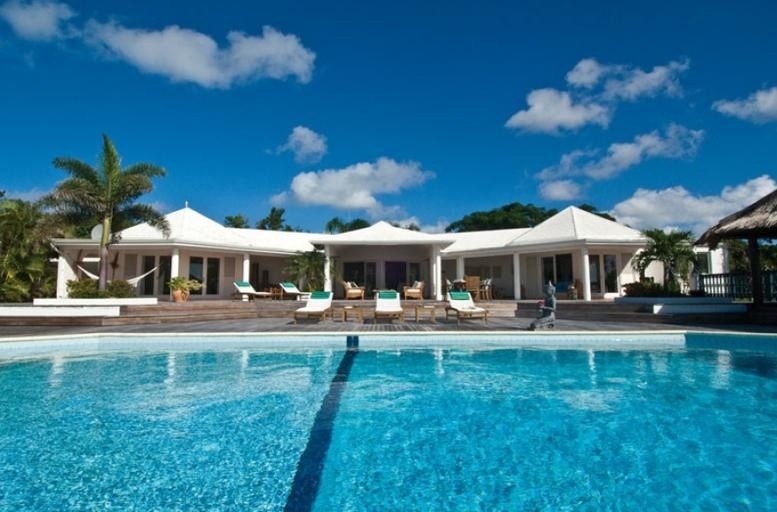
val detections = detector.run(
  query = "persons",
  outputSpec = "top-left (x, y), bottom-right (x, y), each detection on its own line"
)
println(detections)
top-left (530, 286), bottom-right (557, 330)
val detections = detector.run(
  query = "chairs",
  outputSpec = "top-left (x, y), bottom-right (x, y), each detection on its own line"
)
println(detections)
top-left (444, 275), bottom-right (493, 302)
top-left (342, 280), bottom-right (365, 298)
top-left (280, 282), bottom-right (334, 322)
top-left (445, 291), bottom-right (488, 326)
top-left (233, 281), bottom-right (271, 301)
top-left (375, 290), bottom-right (403, 324)
top-left (403, 280), bottom-right (425, 299)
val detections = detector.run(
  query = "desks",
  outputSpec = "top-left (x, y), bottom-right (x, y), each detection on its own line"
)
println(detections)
top-left (415, 305), bottom-right (437, 323)
top-left (342, 305), bottom-right (364, 322)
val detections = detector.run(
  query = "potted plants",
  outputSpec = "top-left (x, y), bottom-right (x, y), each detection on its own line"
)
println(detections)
top-left (165, 276), bottom-right (205, 302)
